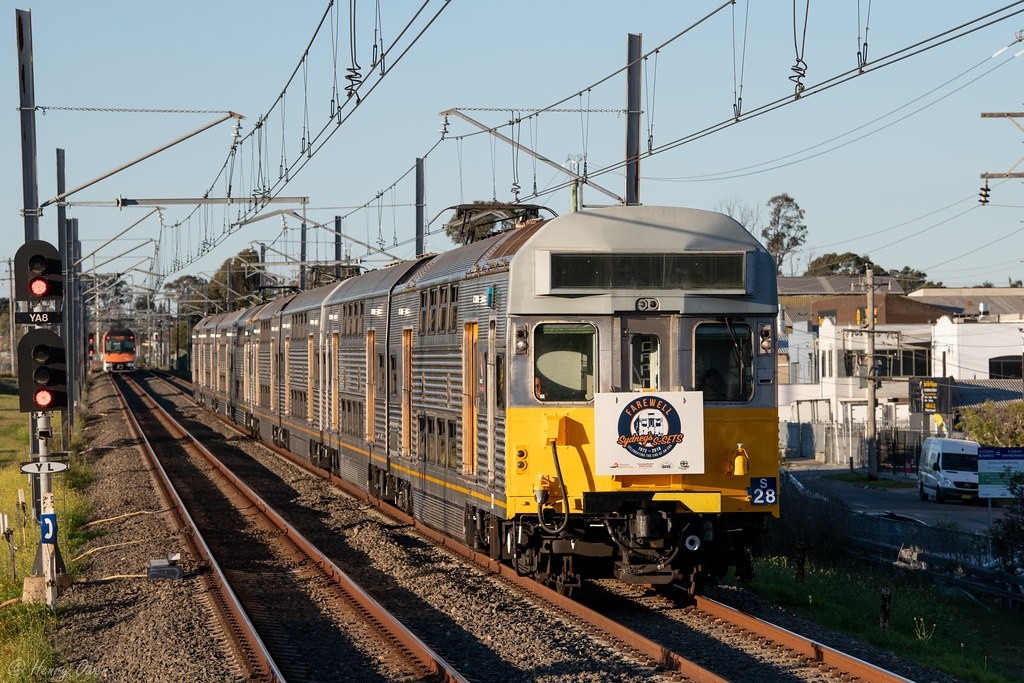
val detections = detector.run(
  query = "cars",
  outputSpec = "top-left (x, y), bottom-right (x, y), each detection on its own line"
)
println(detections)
top-left (915, 437), bottom-right (981, 502)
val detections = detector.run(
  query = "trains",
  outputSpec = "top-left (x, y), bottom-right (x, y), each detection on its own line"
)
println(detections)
top-left (102, 324), bottom-right (141, 371)
top-left (189, 201), bottom-right (778, 583)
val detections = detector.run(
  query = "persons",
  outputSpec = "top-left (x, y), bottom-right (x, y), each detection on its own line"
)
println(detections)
top-left (535, 376), bottom-right (549, 401)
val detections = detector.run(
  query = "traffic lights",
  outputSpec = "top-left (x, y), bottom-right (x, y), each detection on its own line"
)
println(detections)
top-left (14, 237), bottom-right (61, 303)
top-left (15, 327), bottom-right (67, 413)
